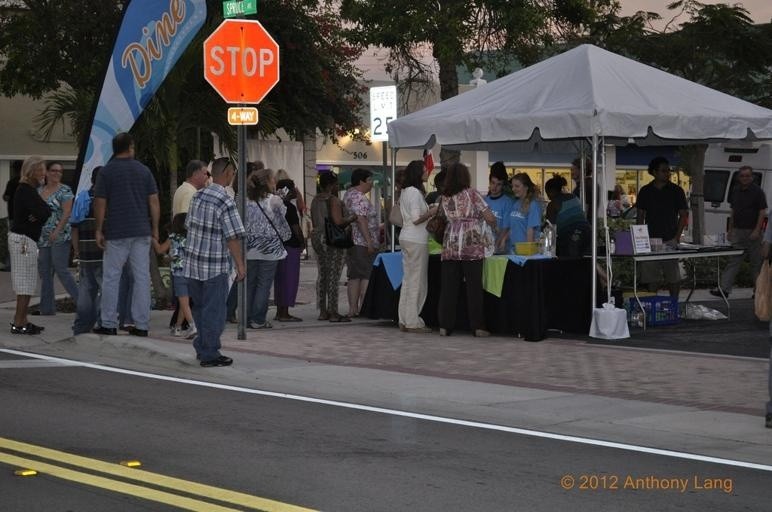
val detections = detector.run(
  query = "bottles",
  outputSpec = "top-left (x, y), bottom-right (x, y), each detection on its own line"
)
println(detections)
top-left (610, 239), bottom-right (616, 254)
top-left (537, 231), bottom-right (546, 255)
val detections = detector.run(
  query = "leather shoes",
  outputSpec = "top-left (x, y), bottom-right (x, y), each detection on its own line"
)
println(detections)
top-left (710, 290), bottom-right (728, 298)
top-left (200, 355), bottom-right (233, 366)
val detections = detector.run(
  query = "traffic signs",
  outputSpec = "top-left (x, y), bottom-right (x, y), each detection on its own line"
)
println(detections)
top-left (370, 86), bottom-right (397, 141)
top-left (228, 107), bottom-right (259, 126)
top-left (223, 0), bottom-right (256, 19)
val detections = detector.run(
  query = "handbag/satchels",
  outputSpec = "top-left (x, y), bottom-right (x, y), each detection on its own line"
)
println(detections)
top-left (754, 259), bottom-right (771, 322)
top-left (324, 196), bottom-right (354, 249)
top-left (425, 198), bottom-right (447, 244)
top-left (588, 296), bottom-right (631, 340)
top-left (389, 198), bottom-right (402, 226)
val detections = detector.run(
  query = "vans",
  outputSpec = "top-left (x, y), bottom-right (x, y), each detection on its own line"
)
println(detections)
top-left (684, 141), bottom-right (772, 246)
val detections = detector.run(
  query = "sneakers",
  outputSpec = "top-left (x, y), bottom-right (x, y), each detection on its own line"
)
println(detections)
top-left (475, 324), bottom-right (491, 336)
top-left (11, 310), bottom-right (360, 339)
top-left (399, 323), bottom-right (406, 330)
top-left (406, 326), bottom-right (432, 332)
top-left (439, 327), bottom-right (448, 335)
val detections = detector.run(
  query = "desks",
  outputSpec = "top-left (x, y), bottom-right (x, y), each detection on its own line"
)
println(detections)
top-left (358, 251), bottom-right (603, 341)
top-left (583, 250), bottom-right (745, 336)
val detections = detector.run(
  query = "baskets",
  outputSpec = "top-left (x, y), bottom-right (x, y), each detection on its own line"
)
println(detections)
top-left (630, 296), bottom-right (678, 326)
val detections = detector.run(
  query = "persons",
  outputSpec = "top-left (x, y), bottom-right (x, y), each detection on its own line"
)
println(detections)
top-left (0, 132), bottom-right (772, 428)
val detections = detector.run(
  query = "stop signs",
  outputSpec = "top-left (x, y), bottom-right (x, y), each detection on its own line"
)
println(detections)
top-left (202, 20), bottom-right (279, 104)
